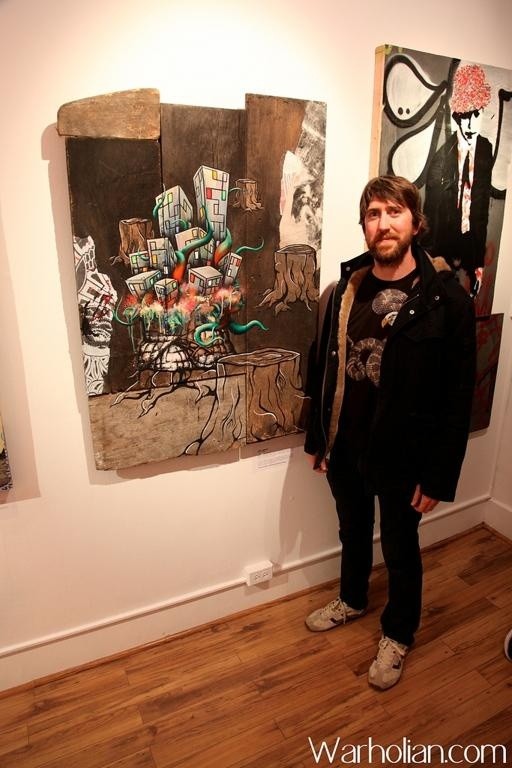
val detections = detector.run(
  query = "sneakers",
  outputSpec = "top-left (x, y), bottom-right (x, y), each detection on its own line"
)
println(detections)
top-left (306, 596), bottom-right (369, 631)
top-left (368, 633), bottom-right (409, 690)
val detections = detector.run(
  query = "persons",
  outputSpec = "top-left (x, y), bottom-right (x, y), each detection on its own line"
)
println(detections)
top-left (302, 175), bottom-right (479, 693)
top-left (420, 64), bottom-right (493, 303)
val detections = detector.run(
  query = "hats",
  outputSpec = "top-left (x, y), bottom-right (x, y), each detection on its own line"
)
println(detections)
top-left (451, 64), bottom-right (490, 113)
